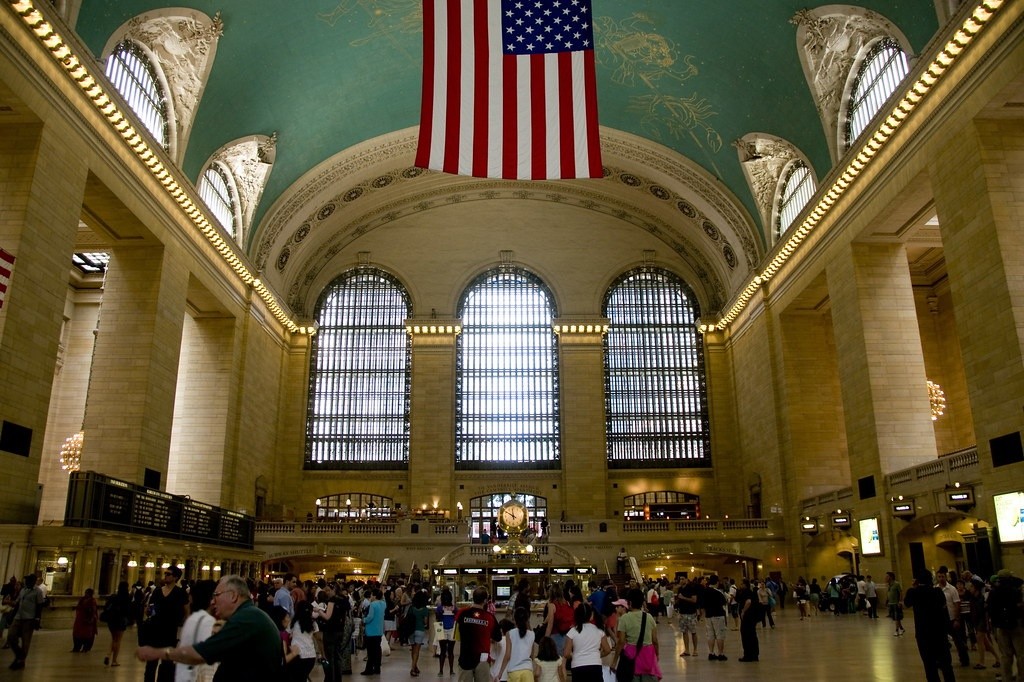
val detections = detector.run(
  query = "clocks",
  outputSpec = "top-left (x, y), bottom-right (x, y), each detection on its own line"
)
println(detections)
top-left (498, 498), bottom-right (530, 534)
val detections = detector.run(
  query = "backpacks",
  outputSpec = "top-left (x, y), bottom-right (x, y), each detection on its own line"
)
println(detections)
top-left (353, 587), bottom-right (363, 602)
top-left (730, 586), bottom-right (741, 603)
top-left (134, 587), bottom-right (144, 604)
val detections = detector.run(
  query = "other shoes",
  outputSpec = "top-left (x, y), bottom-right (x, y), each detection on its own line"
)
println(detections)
top-left (8, 649), bottom-right (25, 671)
top-left (900, 629), bottom-right (905, 635)
top-left (708, 653), bottom-right (718, 661)
top-left (104, 657), bottom-right (110, 666)
top-left (738, 655), bottom-right (759, 662)
top-left (800, 616), bottom-right (808, 621)
top-left (2, 642), bottom-right (9, 649)
top-left (111, 662), bottom-right (121, 666)
top-left (361, 668), bottom-right (380, 676)
top-left (447, 673), bottom-right (455, 680)
top-left (718, 654), bottom-right (727, 661)
top-left (410, 669), bottom-right (420, 677)
top-left (971, 646), bottom-right (977, 651)
top-left (893, 632), bottom-right (899, 636)
top-left (341, 670), bottom-right (352, 675)
top-left (435, 673), bottom-right (444, 679)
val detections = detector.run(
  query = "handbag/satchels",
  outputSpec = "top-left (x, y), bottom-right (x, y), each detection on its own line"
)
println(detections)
top-left (433, 621), bottom-right (445, 641)
top-left (192, 614), bottom-right (221, 682)
top-left (316, 607), bottom-right (328, 632)
top-left (652, 591), bottom-right (660, 607)
top-left (614, 649), bottom-right (635, 682)
top-left (666, 601), bottom-right (675, 619)
top-left (399, 605), bottom-right (416, 641)
top-left (380, 633), bottom-right (391, 656)
top-left (864, 599), bottom-right (872, 609)
top-left (98, 595), bottom-right (114, 623)
top-left (0, 595), bottom-right (12, 613)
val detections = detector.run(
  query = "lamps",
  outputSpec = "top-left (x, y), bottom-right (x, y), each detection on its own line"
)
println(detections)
top-left (127, 549), bottom-right (138, 567)
top-left (201, 559), bottom-right (210, 571)
top-left (56, 550), bottom-right (71, 565)
top-left (161, 552), bottom-right (171, 569)
top-left (346, 498), bottom-right (352, 517)
top-left (926, 381), bottom-right (946, 421)
top-left (525, 545), bottom-right (533, 553)
top-left (493, 544), bottom-right (501, 555)
top-left (213, 559), bottom-right (222, 572)
top-left (145, 553), bottom-right (155, 568)
top-left (176, 555), bottom-right (186, 569)
top-left (59, 255), bottom-right (110, 475)
top-left (316, 498), bottom-right (321, 517)
top-left (457, 502), bottom-right (464, 519)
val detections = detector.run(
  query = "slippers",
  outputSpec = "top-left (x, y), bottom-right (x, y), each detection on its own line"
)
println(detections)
top-left (973, 664), bottom-right (986, 670)
top-left (692, 653), bottom-right (698, 657)
top-left (680, 653), bottom-right (690, 657)
top-left (993, 662), bottom-right (1000, 667)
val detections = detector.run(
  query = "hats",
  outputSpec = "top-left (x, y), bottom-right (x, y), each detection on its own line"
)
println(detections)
top-left (267, 586), bottom-right (276, 598)
top-left (990, 575), bottom-right (1001, 583)
top-left (23, 574), bottom-right (37, 581)
top-left (997, 569), bottom-right (1015, 578)
top-left (611, 599), bottom-right (628, 610)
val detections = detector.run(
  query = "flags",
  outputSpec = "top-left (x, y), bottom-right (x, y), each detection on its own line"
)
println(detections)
top-left (414, 0), bottom-right (604, 179)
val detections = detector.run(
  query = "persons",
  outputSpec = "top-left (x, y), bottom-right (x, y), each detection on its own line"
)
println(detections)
top-left (492, 535), bottom-right (499, 545)
top-left (617, 548), bottom-right (628, 574)
top-left (738, 578), bottom-right (759, 661)
top-left (885, 572), bottom-right (905, 636)
top-left (101, 563), bottom-right (680, 682)
top-left (482, 529), bottom-right (489, 544)
top-left (69, 589), bottom-right (99, 653)
top-left (0, 573), bottom-right (46, 670)
top-left (490, 519), bottom-right (504, 538)
top-left (790, 573), bottom-right (880, 620)
top-left (621, 576), bottom-right (788, 624)
top-left (541, 518), bottom-right (549, 536)
top-left (675, 575), bottom-right (698, 657)
top-left (902, 566), bottom-right (1024, 682)
top-left (700, 574), bottom-right (730, 660)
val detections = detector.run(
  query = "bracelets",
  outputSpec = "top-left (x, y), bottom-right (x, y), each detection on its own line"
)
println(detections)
top-left (899, 602), bottom-right (902, 604)
top-left (166, 647), bottom-right (170, 660)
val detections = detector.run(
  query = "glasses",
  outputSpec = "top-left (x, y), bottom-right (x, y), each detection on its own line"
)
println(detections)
top-left (213, 590), bottom-right (234, 604)
top-left (267, 593), bottom-right (270, 598)
top-left (164, 572), bottom-right (173, 576)
